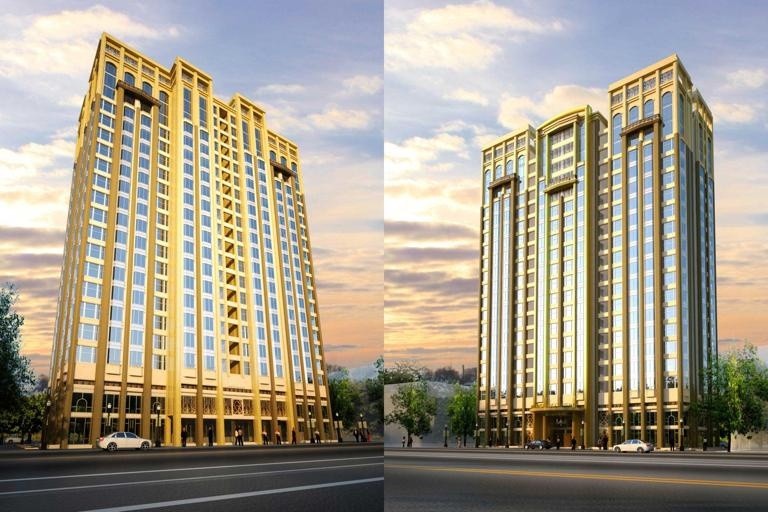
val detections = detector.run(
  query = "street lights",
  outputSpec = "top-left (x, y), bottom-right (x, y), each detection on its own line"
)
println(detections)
top-left (333, 411), bottom-right (343, 443)
top-left (581, 419), bottom-right (585, 450)
top-left (679, 415), bottom-right (684, 451)
top-left (622, 419), bottom-right (626, 442)
top-left (474, 423), bottom-right (481, 448)
top-left (506, 421), bottom-right (510, 448)
top-left (107, 402), bottom-right (112, 433)
top-left (44, 395), bottom-right (52, 450)
top-left (358, 411), bottom-right (368, 441)
top-left (442, 424), bottom-right (449, 447)
top-left (156, 403), bottom-right (161, 447)
top-left (307, 411), bottom-right (316, 443)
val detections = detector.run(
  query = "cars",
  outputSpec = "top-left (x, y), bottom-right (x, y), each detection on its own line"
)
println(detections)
top-left (704, 441), bottom-right (728, 450)
top-left (4, 436), bottom-right (28, 444)
top-left (612, 438), bottom-right (654, 453)
top-left (95, 431), bottom-right (153, 452)
top-left (524, 439), bottom-right (552, 451)
